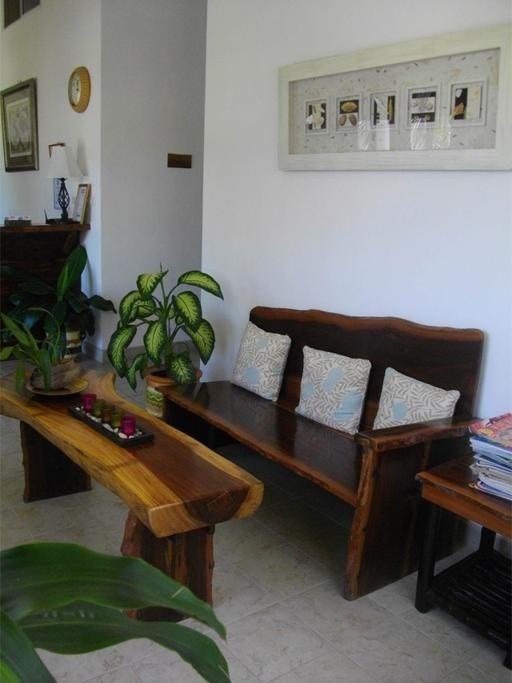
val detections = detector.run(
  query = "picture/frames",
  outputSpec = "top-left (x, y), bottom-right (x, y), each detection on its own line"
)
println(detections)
top-left (0, 79), bottom-right (39, 173)
top-left (277, 23), bottom-right (512, 171)
top-left (72, 184), bottom-right (91, 224)
top-left (48, 143), bottom-right (65, 158)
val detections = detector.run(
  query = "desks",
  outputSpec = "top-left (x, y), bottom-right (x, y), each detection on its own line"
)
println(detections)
top-left (0, 361), bottom-right (263, 623)
top-left (0, 223), bottom-right (90, 347)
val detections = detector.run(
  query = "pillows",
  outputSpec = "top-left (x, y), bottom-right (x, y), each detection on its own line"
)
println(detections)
top-left (230, 321), bottom-right (291, 401)
top-left (372, 367), bottom-right (460, 430)
top-left (295, 346), bottom-right (372, 436)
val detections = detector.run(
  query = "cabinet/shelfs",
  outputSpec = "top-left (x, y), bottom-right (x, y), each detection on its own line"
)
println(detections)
top-left (416, 454), bottom-right (512, 671)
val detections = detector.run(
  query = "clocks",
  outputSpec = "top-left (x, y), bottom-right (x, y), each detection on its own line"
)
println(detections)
top-left (68, 67), bottom-right (91, 113)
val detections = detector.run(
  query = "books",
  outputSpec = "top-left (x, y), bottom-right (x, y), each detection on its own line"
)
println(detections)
top-left (468, 412), bottom-right (512, 502)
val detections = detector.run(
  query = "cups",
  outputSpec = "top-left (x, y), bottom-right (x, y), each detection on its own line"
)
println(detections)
top-left (83, 394), bottom-right (136, 437)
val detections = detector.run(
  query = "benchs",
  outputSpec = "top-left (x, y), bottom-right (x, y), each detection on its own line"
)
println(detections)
top-left (154, 307), bottom-right (484, 602)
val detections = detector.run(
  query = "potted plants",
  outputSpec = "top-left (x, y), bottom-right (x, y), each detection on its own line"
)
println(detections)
top-left (0, 303), bottom-right (80, 390)
top-left (23, 246), bottom-right (117, 360)
top-left (107, 263), bottom-right (224, 418)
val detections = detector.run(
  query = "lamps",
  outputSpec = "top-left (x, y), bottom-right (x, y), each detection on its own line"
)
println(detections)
top-left (46, 146), bottom-right (83, 224)
